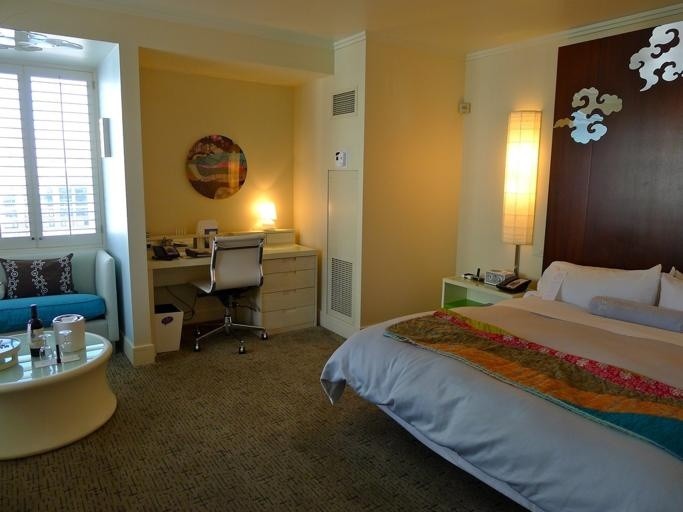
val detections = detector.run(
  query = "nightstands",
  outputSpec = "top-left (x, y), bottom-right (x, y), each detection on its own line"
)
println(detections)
top-left (440, 273), bottom-right (535, 309)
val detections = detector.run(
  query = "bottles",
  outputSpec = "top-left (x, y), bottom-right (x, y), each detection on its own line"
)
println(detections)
top-left (146, 232), bottom-right (150, 248)
top-left (26, 303), bottom-right (45, 356)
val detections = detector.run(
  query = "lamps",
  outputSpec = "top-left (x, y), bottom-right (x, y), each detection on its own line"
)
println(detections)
top-left (502, 110), bottom-right (541, 274)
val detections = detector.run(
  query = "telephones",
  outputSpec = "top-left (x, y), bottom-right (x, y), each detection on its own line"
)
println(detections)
top-left (497, 276), bottom-right (532, 294)
top-left (152, 245), bottom-right (180, 260)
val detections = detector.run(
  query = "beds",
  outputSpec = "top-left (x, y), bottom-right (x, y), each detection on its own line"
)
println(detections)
top-left (319, 288), bottom-right (683, 512)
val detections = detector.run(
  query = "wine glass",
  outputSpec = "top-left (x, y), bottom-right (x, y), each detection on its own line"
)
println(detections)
top-left (37, 333), bottom-right (51, 365)
top-left (60, 330), bottom-right (73, 362)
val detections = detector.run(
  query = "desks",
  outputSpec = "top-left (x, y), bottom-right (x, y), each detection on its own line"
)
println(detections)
top-left (147, 243), bottom-right (318, 339)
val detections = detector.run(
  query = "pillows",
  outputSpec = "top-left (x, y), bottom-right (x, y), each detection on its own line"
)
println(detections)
top-left (586, 295), bottom-right (682, 333)
top-left (535, 259), bottom-right (663, 314)
top-left (668, 266), bottom-right (682, 279)
top-left (658, 271), bottom-right (682, 310)
top-left (0, 253), bottom-right (79, 303)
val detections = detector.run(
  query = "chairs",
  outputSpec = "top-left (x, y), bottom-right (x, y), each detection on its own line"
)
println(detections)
top-left (0, 247), bottom-right (118, 354)
top-left (191, 231), bottom-right (269, 357)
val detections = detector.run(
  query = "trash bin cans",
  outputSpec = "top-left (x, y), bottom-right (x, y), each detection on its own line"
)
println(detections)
top-left (155, 303), bottom-right (184, 353)
